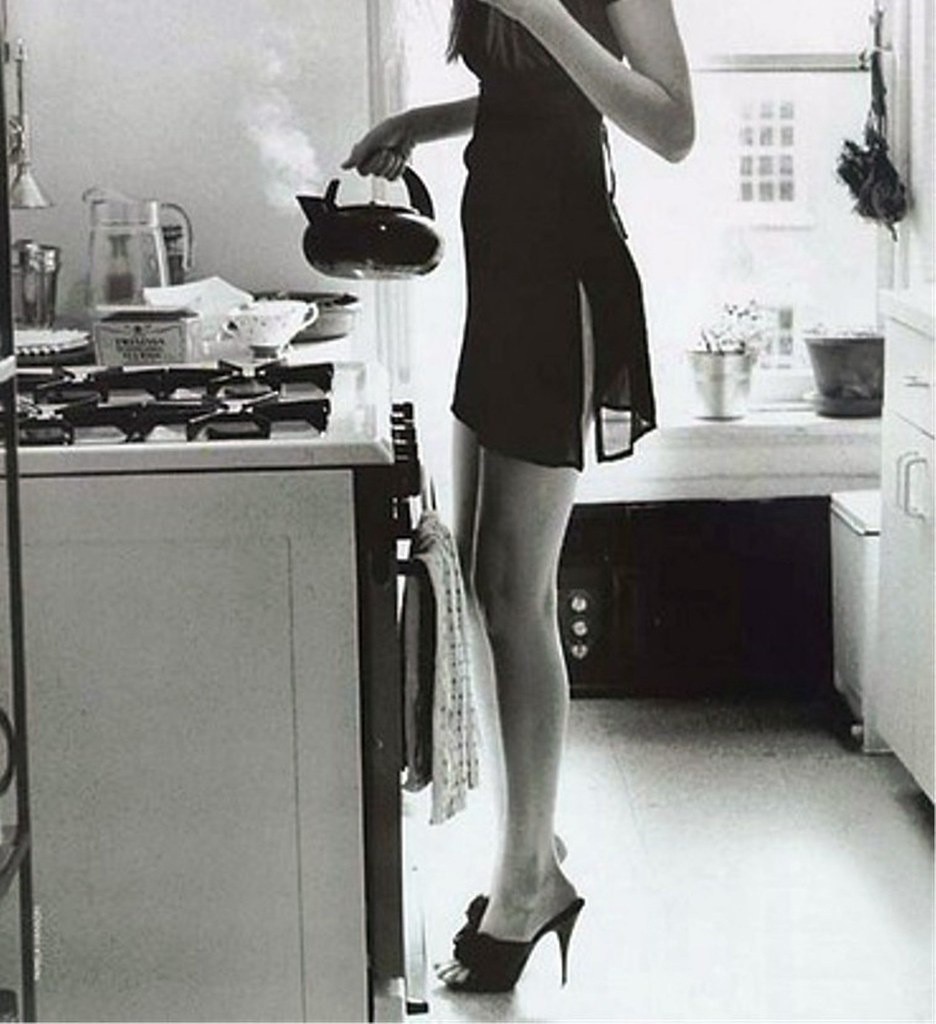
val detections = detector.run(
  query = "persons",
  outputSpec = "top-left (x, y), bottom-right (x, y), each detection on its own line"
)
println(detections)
top-left (341, 0), bottom-right (694, 994)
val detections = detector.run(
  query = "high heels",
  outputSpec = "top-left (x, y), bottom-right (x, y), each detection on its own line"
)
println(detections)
top-left (446, 893), bottom-right (585, 992)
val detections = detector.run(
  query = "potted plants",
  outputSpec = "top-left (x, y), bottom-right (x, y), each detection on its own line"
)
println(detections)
top-left (688, 301), bottom-right (760, 422)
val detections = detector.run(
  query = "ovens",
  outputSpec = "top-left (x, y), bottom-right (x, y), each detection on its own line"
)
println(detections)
top-left (15, 401), bottom-right (437, 1024)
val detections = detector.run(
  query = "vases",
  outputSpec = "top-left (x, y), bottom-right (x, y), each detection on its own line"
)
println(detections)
top-left (807, 336), bottom-right (885, 416)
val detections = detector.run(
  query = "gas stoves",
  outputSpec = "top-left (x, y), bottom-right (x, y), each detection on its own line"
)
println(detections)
top-left (12, 358), bottom-right (397, 475)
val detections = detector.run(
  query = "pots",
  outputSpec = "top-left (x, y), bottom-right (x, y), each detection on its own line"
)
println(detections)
top-left (246, 288), bottom-right (365, 344)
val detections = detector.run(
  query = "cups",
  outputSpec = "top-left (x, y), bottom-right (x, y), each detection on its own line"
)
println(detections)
top-left (802, 336), bottom-right (885, 419)
top-left (220, 301), bottom-right (317, 358)
top-left (11, 240), bottom-right (62, 325)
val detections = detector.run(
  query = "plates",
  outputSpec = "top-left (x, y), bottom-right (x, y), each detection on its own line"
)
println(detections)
top-left (13, 328), bottom-right (90, 354)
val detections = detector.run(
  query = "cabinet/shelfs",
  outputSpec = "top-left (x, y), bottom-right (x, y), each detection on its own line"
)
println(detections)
top-left (558, 495), bottom-right (831, 701)
top-left (864, 291), bottom-right (934, 801)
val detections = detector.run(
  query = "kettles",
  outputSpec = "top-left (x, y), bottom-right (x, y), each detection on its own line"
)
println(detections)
top-left (295, 158), bottom-right (444, 283)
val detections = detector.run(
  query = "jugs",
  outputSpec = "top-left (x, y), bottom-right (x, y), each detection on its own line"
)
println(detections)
top-left (81, 184), bottom-right (195, 330)
